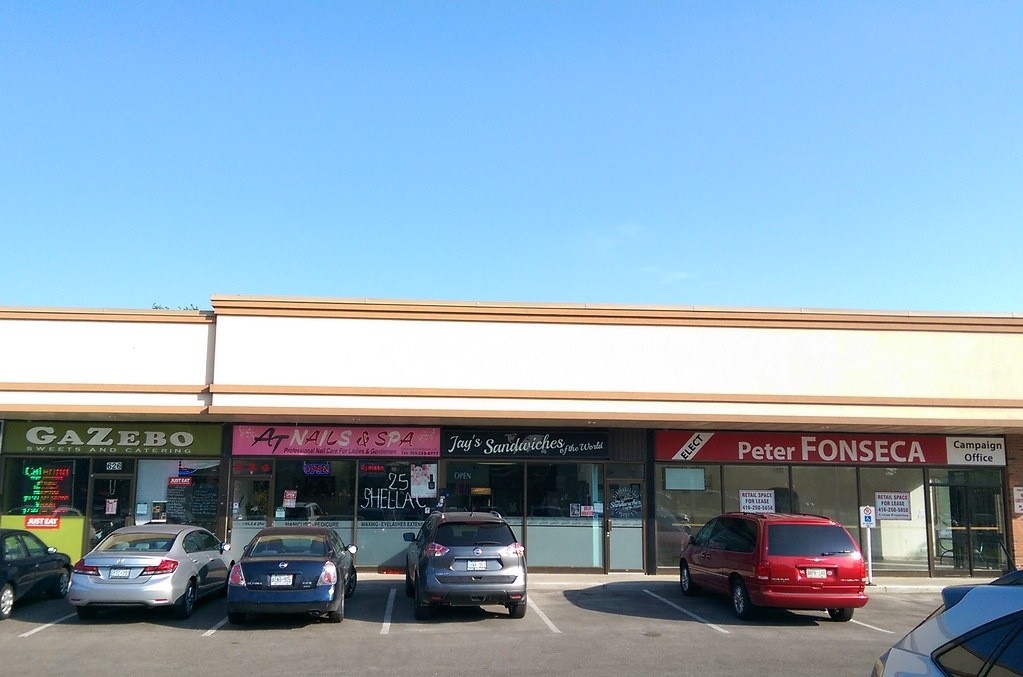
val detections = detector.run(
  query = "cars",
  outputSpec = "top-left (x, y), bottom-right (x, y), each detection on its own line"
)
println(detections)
top-left (68, 523), bottom-right (235, 620)
top-left (871, 570), bottom-right (1023, 677)
top-left (228, 527), bottom-right (357, 622)
top-left (0, 529), bottom-right (70, 619)
top-left (680, 512), bottom-right (868, 622)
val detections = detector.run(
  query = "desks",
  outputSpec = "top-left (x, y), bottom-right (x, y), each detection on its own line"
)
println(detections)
top-left (937, 537), bottom-right (984, 567)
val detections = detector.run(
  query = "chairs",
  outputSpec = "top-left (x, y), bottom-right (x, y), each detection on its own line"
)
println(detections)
top-left (6, 541), bottom-right (16, 560)
top-left (115, 541), bottom-right (129, 548)
top-left (136, 543), bottom-right (151, 549)
top-left (312, 540), bottom-right (323, 553)
top-left (478, 527), bottom-right (498, 540)
top-left (268, 539), bottom-right (283, 551)
top-left (155, 541), bottom-right (167, 548)
top-left (441, 527), bottom-right (454, 543)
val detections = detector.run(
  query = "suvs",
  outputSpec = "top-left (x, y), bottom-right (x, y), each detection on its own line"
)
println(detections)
top-left (402, 511), bottom-right (528, 619)
